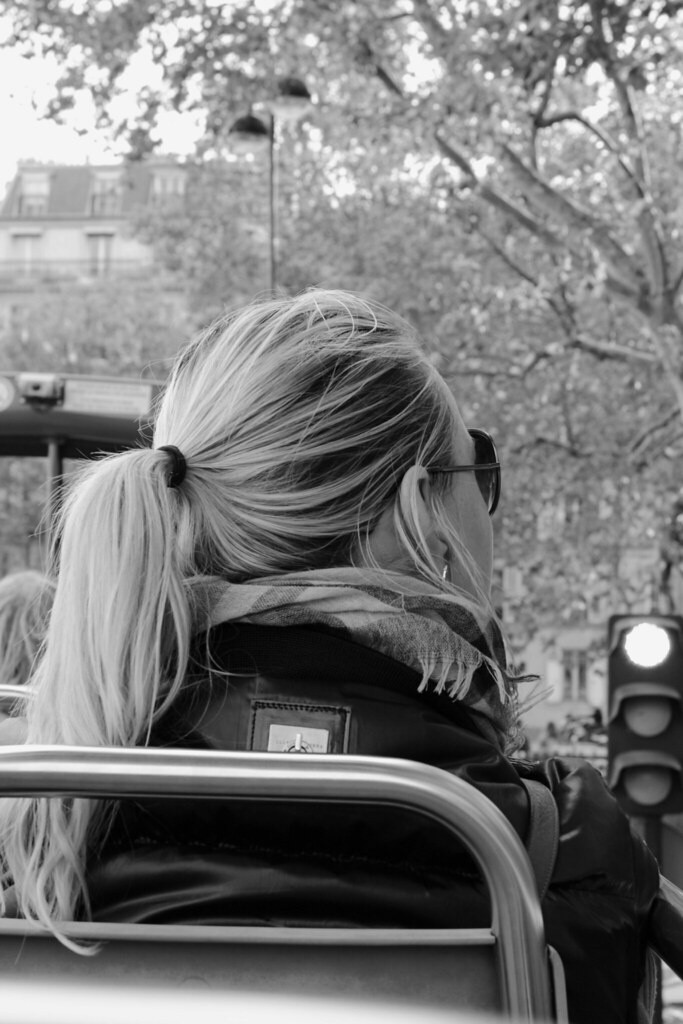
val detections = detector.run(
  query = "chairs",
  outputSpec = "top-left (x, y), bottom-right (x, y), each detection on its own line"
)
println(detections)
top-left (0, 744), bottom-right (568, 1024)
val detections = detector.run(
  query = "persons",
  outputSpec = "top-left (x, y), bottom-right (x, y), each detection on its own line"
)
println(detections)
top-left (0, 287), bottom-right (660, 1023)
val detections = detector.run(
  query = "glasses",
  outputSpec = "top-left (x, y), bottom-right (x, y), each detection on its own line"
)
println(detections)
top-left (362, 428), bottom-right (501, 536)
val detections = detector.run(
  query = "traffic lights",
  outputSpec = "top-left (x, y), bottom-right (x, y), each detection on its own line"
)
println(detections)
top-left (604, 614), bottom-right (683, 822)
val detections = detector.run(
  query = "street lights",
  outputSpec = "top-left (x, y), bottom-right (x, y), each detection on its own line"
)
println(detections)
top-left (225, 74), bottom-right (316, 300)
